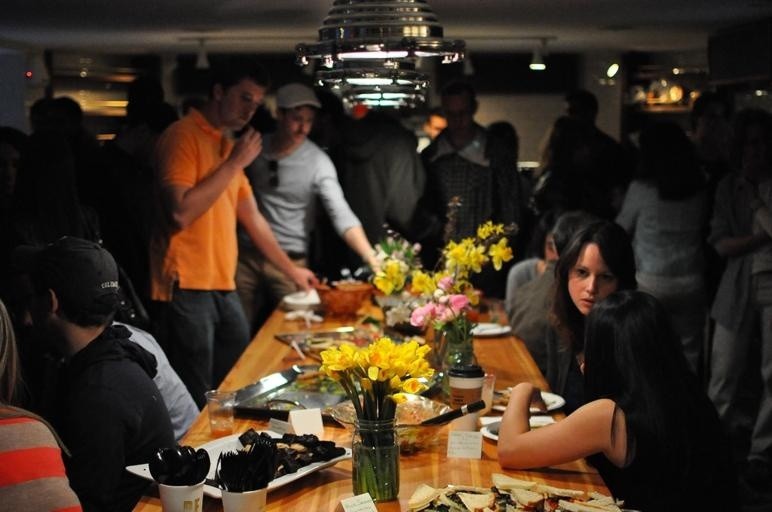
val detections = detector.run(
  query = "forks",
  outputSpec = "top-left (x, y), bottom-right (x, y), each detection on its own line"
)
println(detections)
top-left (213, 439), bottom-right (279, 493)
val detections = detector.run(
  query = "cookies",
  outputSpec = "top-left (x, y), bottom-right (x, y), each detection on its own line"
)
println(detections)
top-left (240, 427), bottom-right (348, 479)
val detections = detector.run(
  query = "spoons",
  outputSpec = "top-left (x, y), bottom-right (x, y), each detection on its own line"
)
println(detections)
top-left (147, 444), bottom-right (210, 485)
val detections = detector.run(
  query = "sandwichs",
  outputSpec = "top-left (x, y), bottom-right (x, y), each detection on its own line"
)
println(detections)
top-left (408, 473), bottom-right (624, 512)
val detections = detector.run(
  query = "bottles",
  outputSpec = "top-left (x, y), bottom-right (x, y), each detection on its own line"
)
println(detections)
top-left (349, 419), bottom-right (398, 502)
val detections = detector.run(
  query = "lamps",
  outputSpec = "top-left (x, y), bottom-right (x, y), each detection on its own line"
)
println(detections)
top-left (293, 0), bottom-right (469, 111)
top-left (180, 33), bottom-right (559, 71)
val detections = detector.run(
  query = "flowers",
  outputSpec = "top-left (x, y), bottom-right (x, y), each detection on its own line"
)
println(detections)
top-left (369, 219), bottom-right (521, 363)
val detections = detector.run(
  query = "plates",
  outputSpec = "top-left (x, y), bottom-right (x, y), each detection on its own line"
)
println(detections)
top-left (284, 290), bottom-right (321, 308)
top-left (472, 320), bottom-right (514, 336)
top-left (480, 419), bottom-right (555, 442)
top-left (492, 388), bottom-right (566, 413)
top-left (122, 431), bottom-right (355, 502)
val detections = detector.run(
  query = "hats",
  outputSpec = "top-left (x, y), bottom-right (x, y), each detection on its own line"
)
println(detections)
top-left (276, 83), bottom-right (321, 109)
top-left (48, 236), bottom-right (120, 315)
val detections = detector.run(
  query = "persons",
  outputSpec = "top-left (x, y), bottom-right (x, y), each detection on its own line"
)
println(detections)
top-left (496, 290), bottom-right (743, 512)
top-left (507, 88), bottom-right (737, 411)
top-left (707, 107), bottom-right (771, 511)
top-left (310, 80), bottom-right (533, 297)
top-left (1, 61), bottom-right (391, 512)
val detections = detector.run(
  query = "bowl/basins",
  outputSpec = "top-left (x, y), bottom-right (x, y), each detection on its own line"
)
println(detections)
top-left (315, 283), bottom-right (373, 317)
top-left (330, 392), bottom-right (452, 455)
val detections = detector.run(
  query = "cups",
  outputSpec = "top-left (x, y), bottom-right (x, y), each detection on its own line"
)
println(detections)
top-left (156, 478), bottom-right (207, 511)
top-left (480, 372), bottom-right (495, 417)
top-left (206, 389), bottom-right (234, 436)
top-left (219, 483), bottom-right (268, 511)
top-left (446, 362), bottom-right (486, 433)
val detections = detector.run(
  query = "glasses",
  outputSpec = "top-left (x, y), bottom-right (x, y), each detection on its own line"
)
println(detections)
top-left (269, 159), bottom-right (278, 187)
